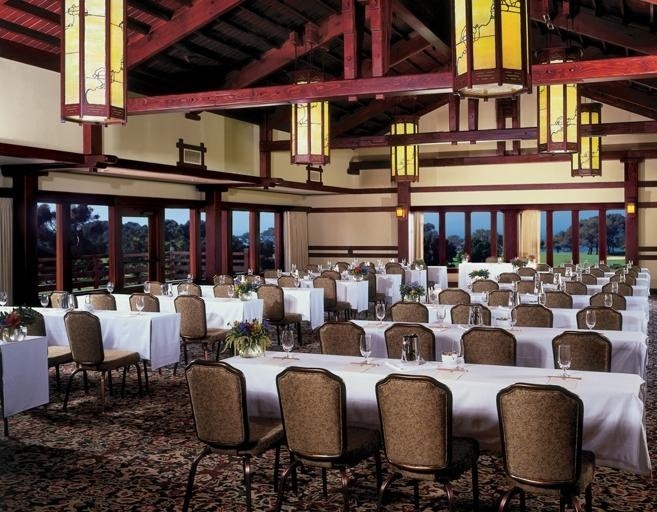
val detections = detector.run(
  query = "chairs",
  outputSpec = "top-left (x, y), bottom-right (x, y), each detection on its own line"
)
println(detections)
top-left (0, 264), bottom-right (651, 475)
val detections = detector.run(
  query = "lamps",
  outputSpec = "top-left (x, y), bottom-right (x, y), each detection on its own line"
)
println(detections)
top-left (450, 0), bottom-right (532, 102)
top-left (624, 199), bottom-right (637, 218)
top-left (61, 0), bottom-right (126, 128)
top-left (536, 14), bottom-right (581, 153)
top-left (290, 41), bottom-right (331, 166)
top-left (569, 96), bottom-right (605, 176)
top-left (396, 205), bottom-right (406, 220)
top-left (389, 95), bottom-right (420, 183)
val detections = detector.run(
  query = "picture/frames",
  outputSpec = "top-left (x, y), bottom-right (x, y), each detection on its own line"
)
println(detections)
top-left (305, 166), bottom-right (323, 186)
top-left (177, 139), bottom-right (207, 170)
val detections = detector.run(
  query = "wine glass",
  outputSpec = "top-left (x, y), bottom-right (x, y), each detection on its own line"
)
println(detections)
top-left (0, 258), bottom-right (634, 378)
